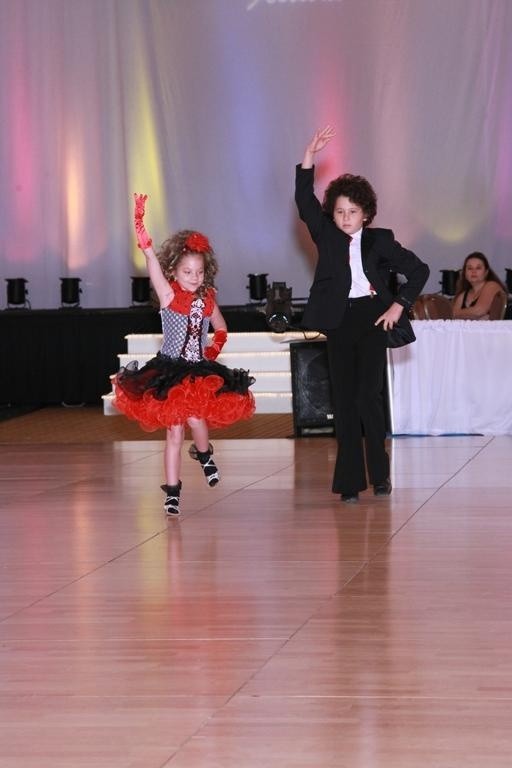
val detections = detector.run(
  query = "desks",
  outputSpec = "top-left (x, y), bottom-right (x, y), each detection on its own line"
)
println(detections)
top-left (384, 318), bottom-right (512, 437)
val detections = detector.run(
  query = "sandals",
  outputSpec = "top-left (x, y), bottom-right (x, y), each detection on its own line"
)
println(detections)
top-left (164, 496), bottom-right (181, 516)
top-left (200, 457), bottom-right (221, 486)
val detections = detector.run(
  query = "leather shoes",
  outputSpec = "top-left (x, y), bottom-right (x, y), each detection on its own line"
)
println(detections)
top-left (374, 476), bottom-right (392, 496)
top-left (341, 492), bottom-right (359, 503)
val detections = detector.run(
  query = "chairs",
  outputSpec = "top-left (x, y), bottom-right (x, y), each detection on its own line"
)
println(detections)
top-left (409, 288), bottom-right (507, 320)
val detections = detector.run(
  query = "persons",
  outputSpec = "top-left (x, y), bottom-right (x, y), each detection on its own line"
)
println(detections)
top-left (452, 250), bottom-right (507, 320)
top-left (113, 193), bottom-right (256, 516)
top-left (296, 125), bottom-right (433, 504)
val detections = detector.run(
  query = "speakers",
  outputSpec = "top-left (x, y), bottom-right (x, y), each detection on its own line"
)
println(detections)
top-left (290, 341), bottom-right (336, 438)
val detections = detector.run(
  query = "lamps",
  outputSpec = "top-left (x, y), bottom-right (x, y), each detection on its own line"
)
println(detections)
top-left (504, 268), bottom-right (512, 294)
top-left (438, 267), bottom-right (463, 297)
top-left (5, 273), bottom-right (269, 311)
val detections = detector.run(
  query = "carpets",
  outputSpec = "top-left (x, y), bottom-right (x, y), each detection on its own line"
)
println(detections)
top-left (0, 400), bottom-right (296, 447)
